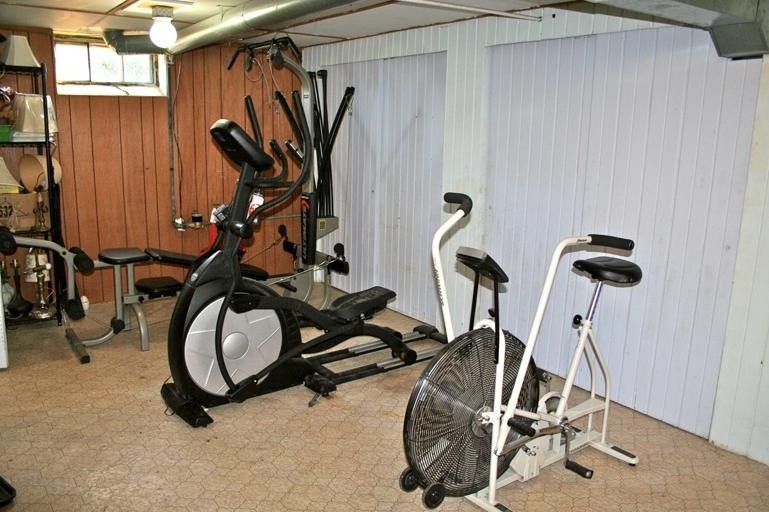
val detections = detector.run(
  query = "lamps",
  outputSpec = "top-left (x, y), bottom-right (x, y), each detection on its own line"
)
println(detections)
top-left (146, 4), bottom-right (180, 52)
top-left (1, 149), bottom-right (61, 319)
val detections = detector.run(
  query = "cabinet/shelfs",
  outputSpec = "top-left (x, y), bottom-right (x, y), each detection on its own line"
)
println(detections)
top-left (0, 61), bottom-right (68, 327)
